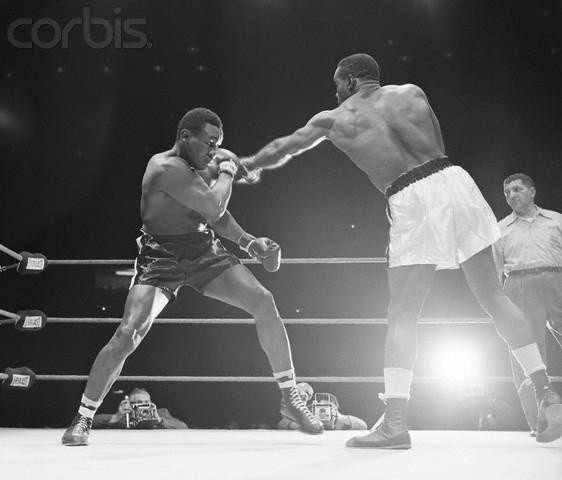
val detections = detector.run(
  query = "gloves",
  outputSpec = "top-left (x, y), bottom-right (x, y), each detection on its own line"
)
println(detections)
top-left (238, 230), bottom-right (282, 273)
top-left (209, 147), bottom-right (249, 185)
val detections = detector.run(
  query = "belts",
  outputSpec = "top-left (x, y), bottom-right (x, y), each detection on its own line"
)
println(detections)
top-left (507, 265), bottom-right (560, 275)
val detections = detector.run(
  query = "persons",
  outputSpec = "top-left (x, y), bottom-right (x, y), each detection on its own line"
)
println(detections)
top-left (60, 105), bottom-right (324, 446)
top-left (276, 391), bottom-right (368, 430)
top-left (230, 53), bottom-right (561, 449)
top-left (90, 385), bottom-right (189, 429)
top-left (491, 173), bottom-right (562, 438)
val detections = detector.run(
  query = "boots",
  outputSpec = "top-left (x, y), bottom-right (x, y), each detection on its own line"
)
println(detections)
top-left (277, 380), bottom-right (324, 433)
top-left (526, 365), bottom-right (561, 442)
top-left (347, 393), bottom-right (411, 450)
top-left (63, 402), bottom-right (96, 447)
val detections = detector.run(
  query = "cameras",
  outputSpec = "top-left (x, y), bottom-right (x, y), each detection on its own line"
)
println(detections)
top-left (308, 393), bottom-right (337, 429)
top-left (125, 395), bottom-right (157, 429)
